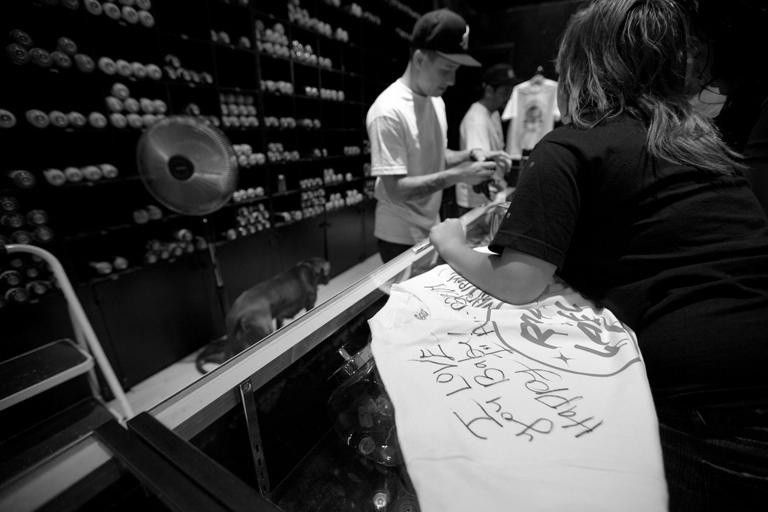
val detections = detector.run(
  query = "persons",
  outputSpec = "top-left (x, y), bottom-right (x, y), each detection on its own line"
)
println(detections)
top-left (429, 2), bottom-right (768, 511)
top-left (365, 7), bottom-right (498, 268)
top-left (452, 64), bottom-right (521, 218)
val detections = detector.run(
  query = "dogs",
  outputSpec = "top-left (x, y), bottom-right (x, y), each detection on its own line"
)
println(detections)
top-left (203, 254), bottom-right (333, 364)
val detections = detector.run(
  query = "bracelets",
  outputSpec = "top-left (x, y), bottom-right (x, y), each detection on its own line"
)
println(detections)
top-left (467, 146), bottom-right (483, 162)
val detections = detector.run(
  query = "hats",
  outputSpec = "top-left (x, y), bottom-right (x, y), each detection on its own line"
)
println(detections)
top-left (411, 9), bottom-right (483, 67)
top-left (482, 62), bottom-right (515, 86)
